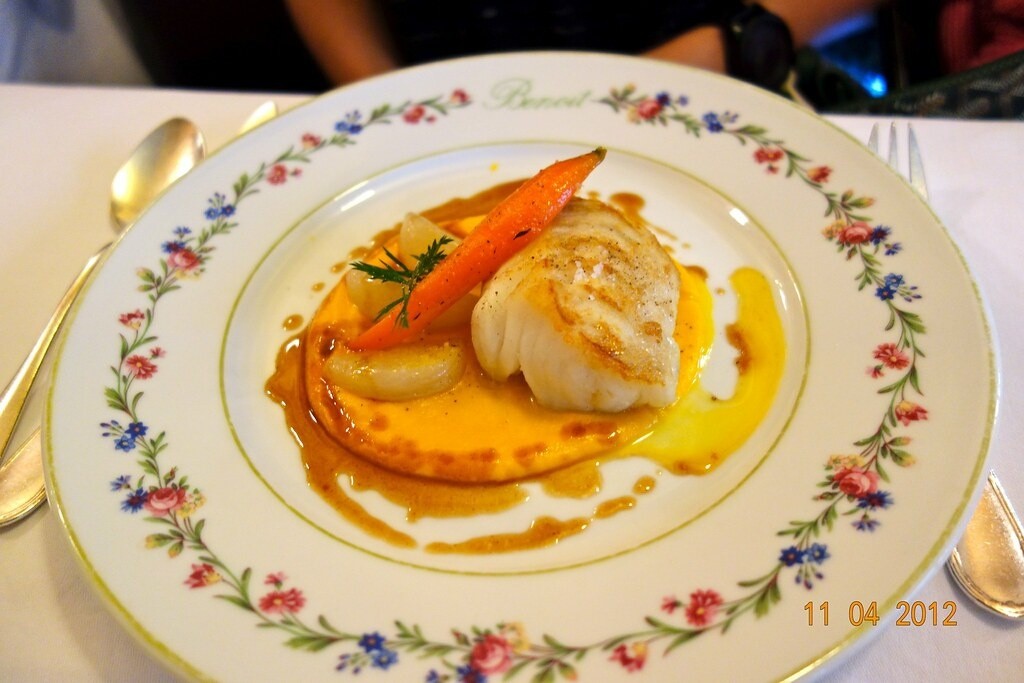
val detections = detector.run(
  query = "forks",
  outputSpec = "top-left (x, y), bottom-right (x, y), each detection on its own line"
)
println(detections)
top-left (863, 121), bottom-right (1024, 621)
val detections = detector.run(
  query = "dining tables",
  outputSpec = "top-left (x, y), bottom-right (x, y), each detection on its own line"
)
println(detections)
top-left (0, 83), bottom-right (1024, 682)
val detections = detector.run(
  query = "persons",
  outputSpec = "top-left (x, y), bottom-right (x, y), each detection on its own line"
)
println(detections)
top-left (117, 0), bottom-right (1024, 124)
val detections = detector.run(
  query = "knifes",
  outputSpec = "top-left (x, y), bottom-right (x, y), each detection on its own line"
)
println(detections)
top-left (2, 96), bottom-right (278, 530)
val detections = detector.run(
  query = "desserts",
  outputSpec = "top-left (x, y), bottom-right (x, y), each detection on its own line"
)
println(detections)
top-left (304, 148), bottom-right (712, 488)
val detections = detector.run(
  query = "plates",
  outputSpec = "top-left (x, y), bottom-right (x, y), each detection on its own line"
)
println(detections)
top-left (40, 50), bottom-right (1002, 683)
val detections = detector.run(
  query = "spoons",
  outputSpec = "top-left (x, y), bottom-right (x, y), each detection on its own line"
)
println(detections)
top-left (1, 118), bottom-right (206, 457)
